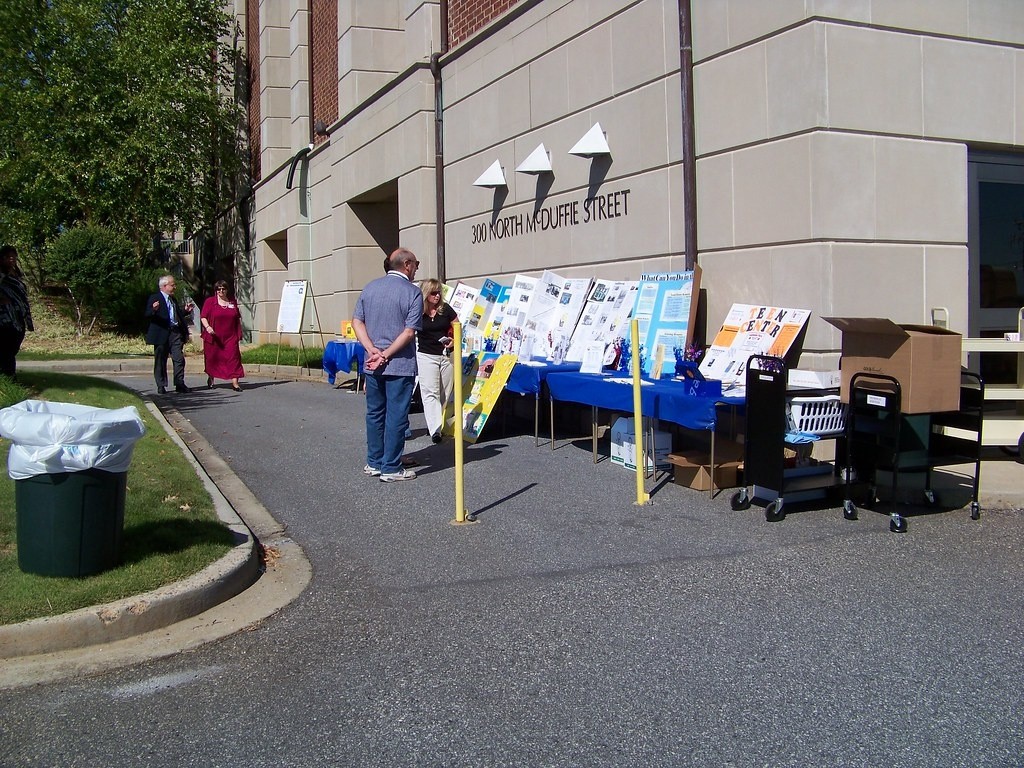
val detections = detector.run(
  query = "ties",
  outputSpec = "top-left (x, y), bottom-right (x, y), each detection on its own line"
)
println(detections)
top-left (168, 296), bottom-right (176, 323)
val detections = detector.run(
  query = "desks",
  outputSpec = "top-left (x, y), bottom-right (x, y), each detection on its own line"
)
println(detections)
top-left (324, 339), bottom-right (367, 394)
top-left (547, 368), bottom-right (753, 502)
top-left (443, 351), bottom-right (584, 448)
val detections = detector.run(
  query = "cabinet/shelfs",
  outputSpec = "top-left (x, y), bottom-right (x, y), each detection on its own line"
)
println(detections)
top-left (731, 353), bottom-right (844, 522)
top-left (918, 306), bottom-right (1024, 459)
top-left (841, 373), bottom-right (990, 534)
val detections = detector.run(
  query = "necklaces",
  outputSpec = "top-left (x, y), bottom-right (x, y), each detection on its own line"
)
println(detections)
top-left (218, 295), bottom-right (228, 309)
top-left (432, 311), bottom-right (436, 321)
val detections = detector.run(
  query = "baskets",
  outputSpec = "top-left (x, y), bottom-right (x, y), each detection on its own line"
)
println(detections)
top-left (790, 394), bottom-right (845, 434)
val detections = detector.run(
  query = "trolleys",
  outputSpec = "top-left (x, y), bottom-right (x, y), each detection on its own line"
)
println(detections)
top-left (841, 371), bottom-right (987, 535)
top-left (729, 355), bottom-right (844, 523)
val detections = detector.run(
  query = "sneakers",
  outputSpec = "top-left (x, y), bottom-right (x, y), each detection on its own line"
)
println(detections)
top-left (379, 467), bottom-right (417, 483)
top-left (364, 464), bottom-right (382, 476)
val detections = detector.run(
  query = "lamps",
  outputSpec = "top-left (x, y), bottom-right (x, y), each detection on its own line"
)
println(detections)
top-left (473, 158), bottom-right (507, 190)
top-left (514, 142), bottom-right (554, 175)
top-left (568, 120), bottom-right (615, 157)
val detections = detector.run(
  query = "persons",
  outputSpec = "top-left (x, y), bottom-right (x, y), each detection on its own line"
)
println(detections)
top-left (614, 431), bottom-right (623, 445)
top-left (146, 275), bottom-right (194, 395)
top-left (200, 279), bottom-right (246, 392)
top-left (352, 247), bottom-right (424, 483)
top-left (628, 437), bottom-right (632, 450)
top-left (415, 278), bottom-right (460, 444)
top-left (0, 245), bottom-right (34, 384)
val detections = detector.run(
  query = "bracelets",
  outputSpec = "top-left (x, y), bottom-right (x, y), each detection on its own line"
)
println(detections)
top-left (205, 325), bottom-right (209, 328)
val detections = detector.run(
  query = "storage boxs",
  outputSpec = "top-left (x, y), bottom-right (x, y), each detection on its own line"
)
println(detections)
top-left (662, 443), bottom-right (748, 492)
top-left (752, 368), bottom-right (840, 389)
top-left (609, 411), bottom-right (654, 464)
top-left (341, 318), bottom-right (361, 340)
top-left (819, 314), bottom-right (961, 414)
top-left (622, 432), bottom-right (672, 472)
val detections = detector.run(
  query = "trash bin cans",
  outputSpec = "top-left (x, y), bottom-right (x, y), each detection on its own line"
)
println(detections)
top-left (3, 399), bottom-right (138, 577)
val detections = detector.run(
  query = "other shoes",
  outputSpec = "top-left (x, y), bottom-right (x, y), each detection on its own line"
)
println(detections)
top-left (432, 433), bottom-right (442, 444)
top-left (176, 384), bottom-right (192, 393)
top-left (232, 385), bottom-right (243, 392)
top-left (405, 433), bottom-right (415, 440)
top-left (157, 386), bottom-right (166, 394)
top-left (207, 379), bottom-right (215, 390)
top-left (401, 455), bottom-right (419, 467)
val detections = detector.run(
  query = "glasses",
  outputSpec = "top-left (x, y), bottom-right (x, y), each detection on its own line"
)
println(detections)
top-left (407, 258), bottom-right (420, 269)
top-left (217, 288), bottom-right (227, 291)
top-left (429, 290), bottom-right (441, 296)
